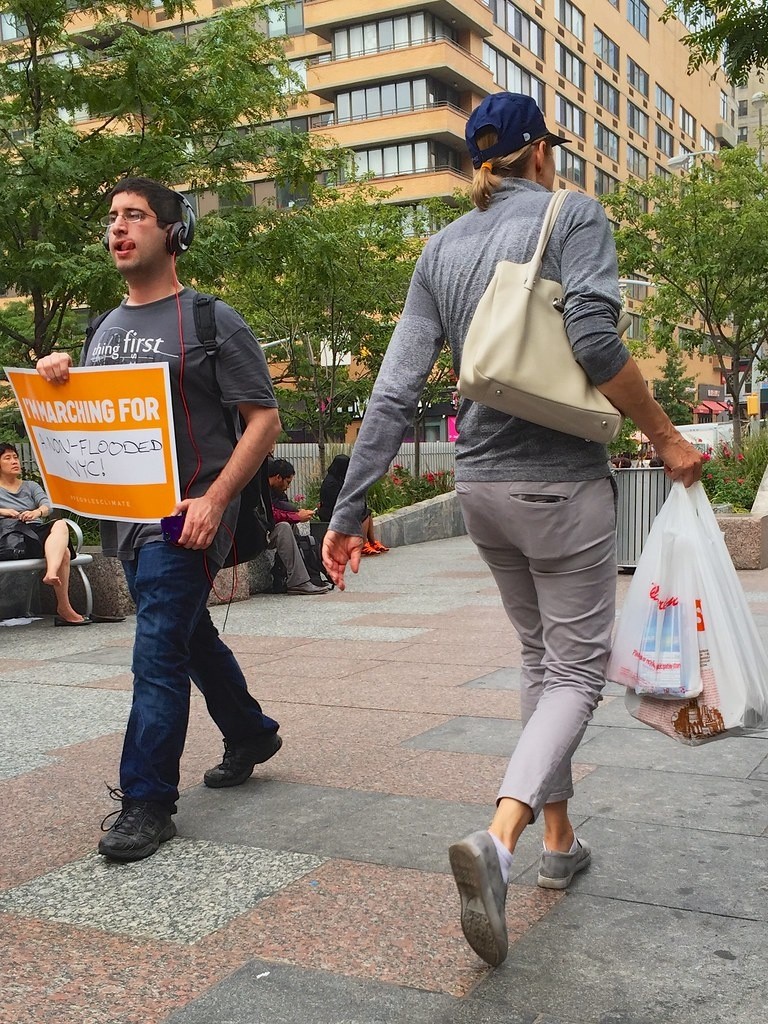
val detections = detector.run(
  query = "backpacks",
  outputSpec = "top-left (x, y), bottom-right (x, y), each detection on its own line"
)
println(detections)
top-left (269, 534), bottom-right (336, 594)
top-left (84, 290), bottom-right (276, 550)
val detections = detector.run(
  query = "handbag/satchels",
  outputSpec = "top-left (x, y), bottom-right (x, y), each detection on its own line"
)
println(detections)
top-left (456, 187), bottom-right (633, 444)
top-left (604, 477), bottom-right (706, 700)
top-left (622, 479), bottom-right (768, 747)
top-left (0, 518), bottom-right (44, 559)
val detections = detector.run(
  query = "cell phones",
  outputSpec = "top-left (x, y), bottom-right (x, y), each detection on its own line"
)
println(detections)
top-left (313, 507), bottom-right (318, 512)
top-left (162, 515), bottom-right (185, 543)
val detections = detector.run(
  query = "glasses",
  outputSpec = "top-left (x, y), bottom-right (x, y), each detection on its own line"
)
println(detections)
top-left (283, 477), bottom-right (291, 485)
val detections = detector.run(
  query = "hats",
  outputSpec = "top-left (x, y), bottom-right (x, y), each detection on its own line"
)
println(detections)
top-left (464, 92), bottom-right (572, 170)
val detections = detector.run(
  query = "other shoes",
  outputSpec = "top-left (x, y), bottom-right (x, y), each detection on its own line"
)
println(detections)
top-left (287, 580), bottom-right (329, 596)
top-left (89, 613), bottom-right (126, 622)
top-left (54, 614), bottom-right (94, 626)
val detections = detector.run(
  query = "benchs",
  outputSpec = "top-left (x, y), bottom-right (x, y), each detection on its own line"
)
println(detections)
top-left (0, 516), bottom-right (95, 623)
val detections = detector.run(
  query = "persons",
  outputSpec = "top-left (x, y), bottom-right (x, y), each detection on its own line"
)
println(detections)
top-left (320, 94), bottom-right (705, 966)
top-left (262, 458), bottom-right (326, 588)
top-left (318, 452), bottom-right (387, 558)
top-left (38, 178), bottom-right (283, 865)
top-left (264, 522), bottom-right (328, 596)
top-left (0, 445), bottom-right (93, 627)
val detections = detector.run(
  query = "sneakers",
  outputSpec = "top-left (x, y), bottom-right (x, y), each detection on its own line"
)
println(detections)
top-left (361, 542), bottom-right (381, 557)
top-left (97, 795), bottom-right (177, 859)
top-left (370, 540), bottom-right (389, 552)
top-left (449, 830), bottom-right (508, 966)
top-left (203, 734), bottom-right (283, 786)
top-left (537, 836), bottom-right (592, 888)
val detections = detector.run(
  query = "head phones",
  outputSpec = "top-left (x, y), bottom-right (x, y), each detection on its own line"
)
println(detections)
top-left (104, 191), bottom-right (195, 255)
